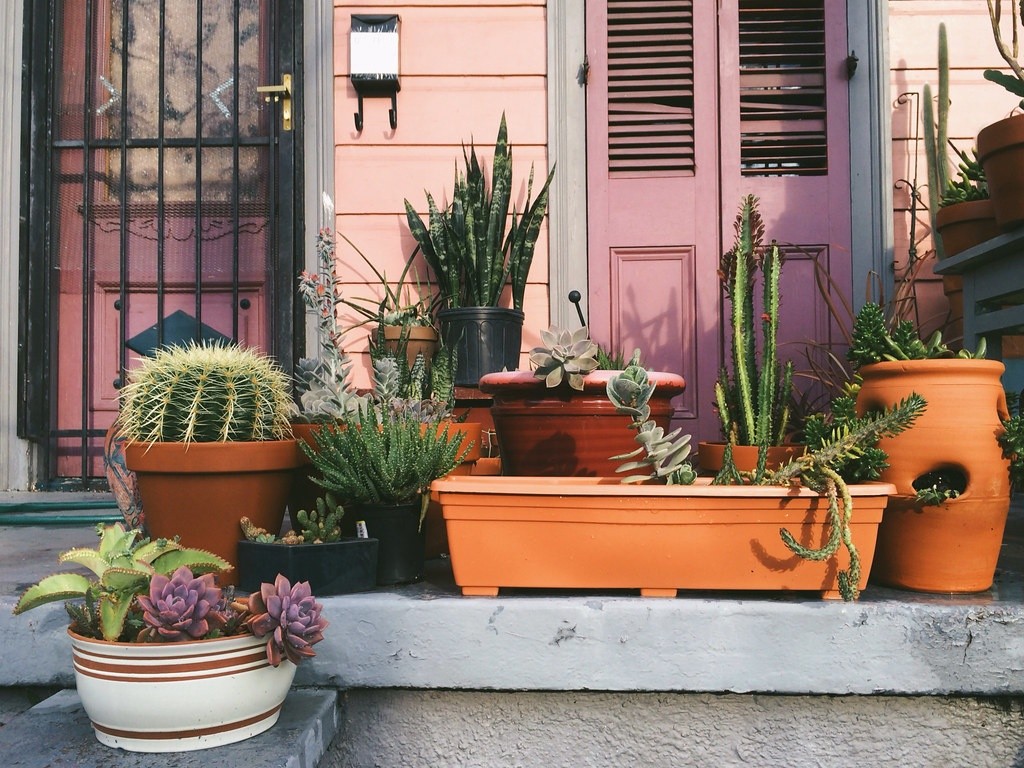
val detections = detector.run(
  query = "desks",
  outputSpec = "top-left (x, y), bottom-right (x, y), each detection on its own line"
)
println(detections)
top-left (932, 228), bottom-right (1024, 418)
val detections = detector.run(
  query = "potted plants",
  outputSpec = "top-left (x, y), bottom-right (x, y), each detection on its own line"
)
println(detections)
top-left (15, 521), bottom-right (330, 754)
top-left (296, 396), bottom-right (474, 585)
top-left (935, 149), bottom-right (1000, 299)
top-left (236, 496), bottom-right (379, 598)
top-left (475, 426), bottom-right (501, 475)
top-left (329, 226), bottom-right (443, 383)
top-left (848, 302), bottom-right (1013, 592)
top-left (479, 326), bottom-right (686, 479)
top-left (976, 0), bottom-right (1024, 228)
top-left (112, 337), bottom-right (300, 588)
top-left (431, 366), bottom-right (930, 601)
top-left (402, 106), bottom-right (557, 387)
top-left (697, 195), bottom-right (811, 477)
